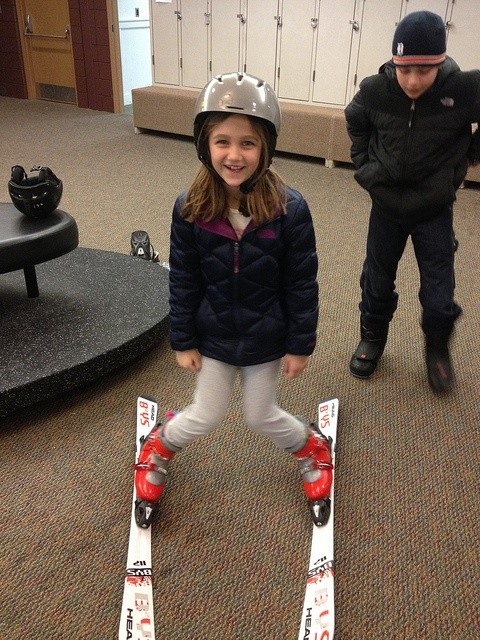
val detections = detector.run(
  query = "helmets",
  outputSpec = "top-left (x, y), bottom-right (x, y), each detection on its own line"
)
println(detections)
top-left (193, 72), bottom-right (281, 138)
top-left (8, 165), bottom-right (63, 218)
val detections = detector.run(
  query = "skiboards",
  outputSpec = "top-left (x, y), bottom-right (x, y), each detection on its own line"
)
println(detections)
top-left (118, 396), bottom-right (339, 639)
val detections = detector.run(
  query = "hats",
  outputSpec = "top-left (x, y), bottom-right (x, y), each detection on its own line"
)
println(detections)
top-left (391, 11), bottom-right (446, 64)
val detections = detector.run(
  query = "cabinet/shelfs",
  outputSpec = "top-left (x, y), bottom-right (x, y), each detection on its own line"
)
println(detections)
top-left (149, 1), bottom-right (180, 87)
top-left (178, 1), bottom-right (209, 88)
top-left (349, 1), bottom-right (404, 114)
top-left (275, 0), bottom-right (317, 102)
top-left (244, 0), bottom-right (280, 93)
top-left (210, 1), bottom-right (243, 83)
top-left (446, 0), bottom-right (480, 75)
top-left (311, 1), bottom-right (359, 112)
top-left (404, 1), bottom-right (450, 34)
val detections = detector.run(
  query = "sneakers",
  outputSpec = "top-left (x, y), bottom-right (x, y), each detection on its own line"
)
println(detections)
top-left (291, 425), bottom-right (333, 500)
top-left (136, 422), bottom-right (180, 500)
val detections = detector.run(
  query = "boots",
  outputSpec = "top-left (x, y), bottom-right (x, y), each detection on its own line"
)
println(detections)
top-left (349, 304), bottom-right (393, 379)
top-left (422, 304), bottom-right (463, 393)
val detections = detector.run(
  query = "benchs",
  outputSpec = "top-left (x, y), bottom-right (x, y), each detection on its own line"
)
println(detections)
top-left (131, 85), bottom-right (351, 168)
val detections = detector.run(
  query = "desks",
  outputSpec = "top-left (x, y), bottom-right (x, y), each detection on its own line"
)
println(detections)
top-left (1, 202), bottom-right (81, 299)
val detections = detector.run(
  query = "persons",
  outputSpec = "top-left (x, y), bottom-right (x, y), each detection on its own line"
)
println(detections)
top-left (341, 9), bottom-right (480, 393)
top-left (130, 71), bottom-right (333, 505)
